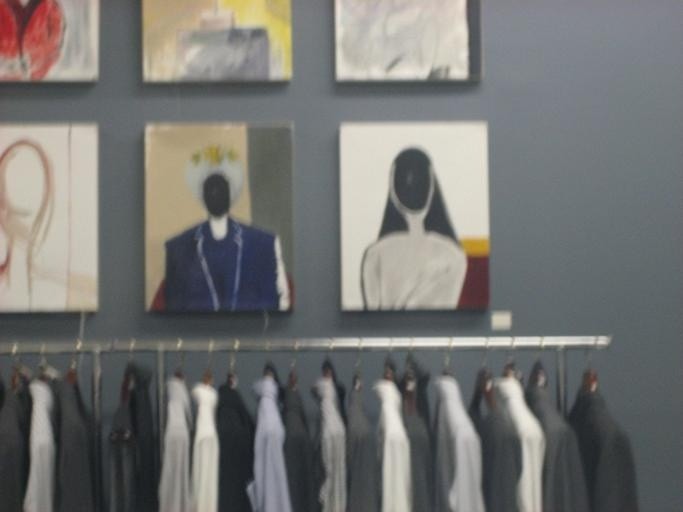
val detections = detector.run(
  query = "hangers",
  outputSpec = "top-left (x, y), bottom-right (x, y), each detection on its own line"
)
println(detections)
top-left (0, 338), bottom-right (614, 449)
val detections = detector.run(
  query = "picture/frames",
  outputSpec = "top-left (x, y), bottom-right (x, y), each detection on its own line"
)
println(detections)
top-left (0, 0), bottom-right (100, 82)
top-left (0, 122), bottom-right (98, 312)
top-left (144, 122), bottom-right (294, 313)
top-left (141, 1), bottom-right (292, 83)
top-left (330, 1), bottom-right (480, 88)
top-left (339, 120), bottom-right (490, 311)
